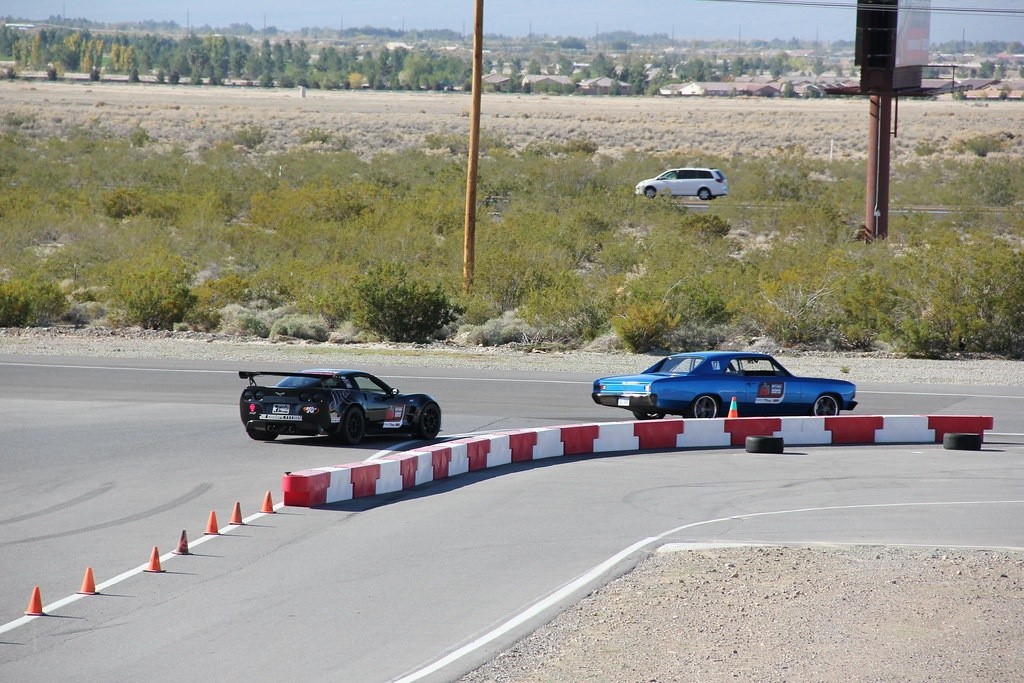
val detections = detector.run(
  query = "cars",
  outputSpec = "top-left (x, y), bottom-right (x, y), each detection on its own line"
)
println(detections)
top-left (595, 355), bottom-right (859, 417)
top-left (635, 167), bottom-right (727, 201)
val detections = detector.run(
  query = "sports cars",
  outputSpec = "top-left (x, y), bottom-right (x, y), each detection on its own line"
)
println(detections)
top-left (242, 366), bottom-right (442, 444)
